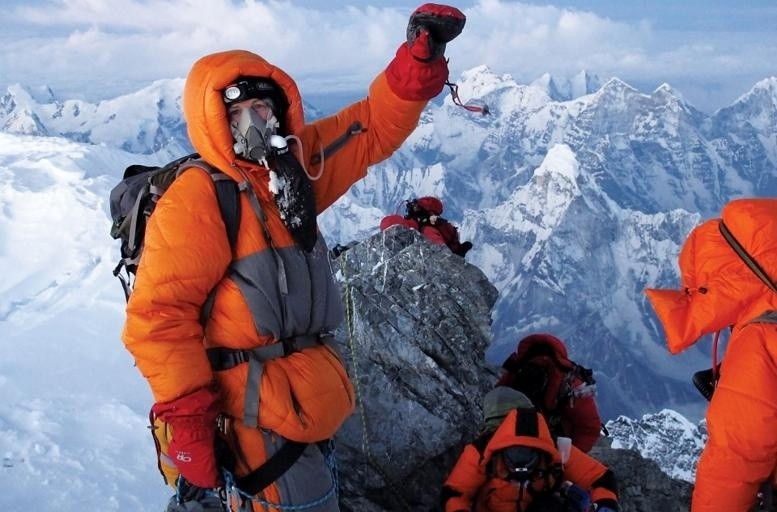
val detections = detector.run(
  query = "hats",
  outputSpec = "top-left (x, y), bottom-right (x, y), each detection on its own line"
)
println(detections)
top-left (219, 74), bottom-right (288, 118)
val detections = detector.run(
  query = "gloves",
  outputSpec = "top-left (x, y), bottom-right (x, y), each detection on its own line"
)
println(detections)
top-left (151, 388), bottom-right (228, 488)
top-left (385, 3), bottom-right (465, 100)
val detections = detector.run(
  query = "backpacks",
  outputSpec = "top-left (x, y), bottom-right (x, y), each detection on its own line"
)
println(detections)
top-left (111, 152), bottom-right (240, 323)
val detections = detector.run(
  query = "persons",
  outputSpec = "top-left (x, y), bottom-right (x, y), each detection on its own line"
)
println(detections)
top-left (440, 407), bottom-right (621, 512)
top-left (645, 196), bottom-right (777, 512)
top-left (121, 3), bottom-right (489, 511)
top-left (379, 195), bottom-right (473, 258)
top-left (495, 334), bottom-right (601, 453)
